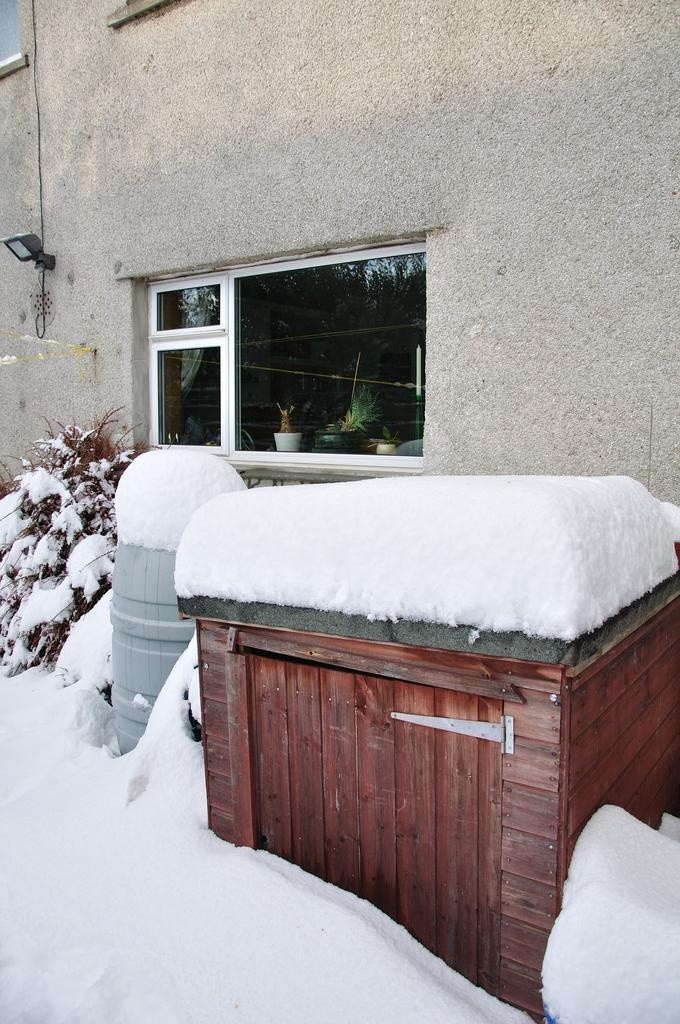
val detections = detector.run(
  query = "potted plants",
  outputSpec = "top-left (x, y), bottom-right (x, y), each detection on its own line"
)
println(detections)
top-left (274, 403), bottom-right (303, 451)
top-left (369, 425), bottom-right (400, 454)
top-left (313, 383), bottom-right (384, 449)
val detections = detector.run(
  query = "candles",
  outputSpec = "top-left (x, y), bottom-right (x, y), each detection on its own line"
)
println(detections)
top-left (416, 343), bottom-right (421, 395)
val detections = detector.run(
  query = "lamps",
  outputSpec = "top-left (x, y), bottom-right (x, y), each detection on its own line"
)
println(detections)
top-left (3, 233), bottom-right (56, 270)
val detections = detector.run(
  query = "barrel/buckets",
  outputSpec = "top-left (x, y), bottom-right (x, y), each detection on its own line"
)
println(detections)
top-left (111, 541), bottom-right (195, 757)
